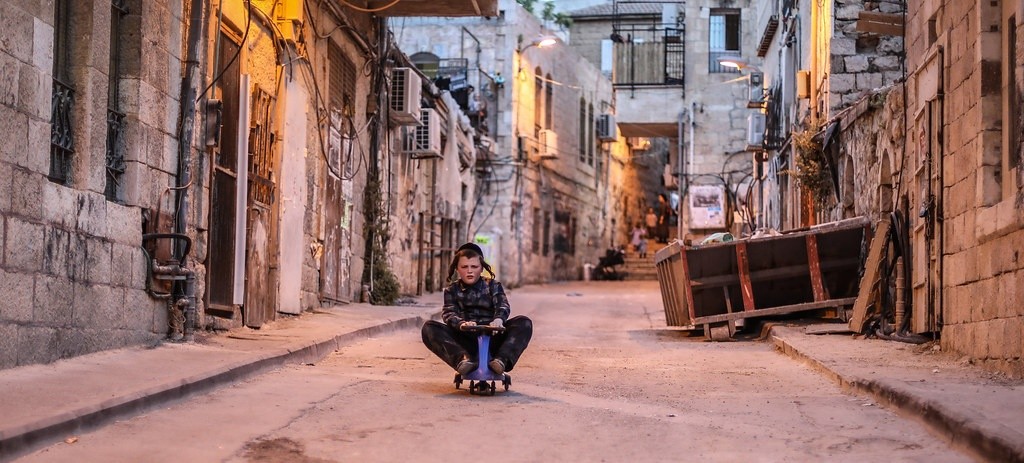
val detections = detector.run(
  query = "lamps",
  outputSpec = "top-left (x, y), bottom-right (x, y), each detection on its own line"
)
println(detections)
top-left (518, 34), bottom-right (556, 55)
top-left (715, 56), bottom-right (765, 79)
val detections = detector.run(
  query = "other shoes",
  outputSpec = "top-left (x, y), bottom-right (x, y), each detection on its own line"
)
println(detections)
top-left (457, 360), bottom-right (477, 375)
top-left (489, 359), bottom-right (505, 374)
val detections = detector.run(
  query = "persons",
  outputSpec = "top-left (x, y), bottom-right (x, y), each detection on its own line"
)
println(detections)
top-left (658, 193), bottom-right (670, 242)
top-left (645, 207), bottom-right (658, 238)
top-left (467, 84), bottom-right (474, 95)
top-left (628, 220), bottom-right (647, 251)
top-left (421, 242), bottom-right (533, 375)
top-left (637, 233), bottom-right (649, 258)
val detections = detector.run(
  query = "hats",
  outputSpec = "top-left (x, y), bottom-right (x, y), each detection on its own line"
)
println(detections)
top-left (459, 243), bottom-right (483, 257)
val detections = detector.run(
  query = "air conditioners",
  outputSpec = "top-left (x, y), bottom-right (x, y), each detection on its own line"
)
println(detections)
top-left (538, 129), bottom-right (559, 160)
top-left (745, 112), bottom-right (766, 151)
top-left (389, 67), bottom-right (422, 126)
top-left (599, 113), bottom-right (618, 143)
top-left (412, 106), bottom-right (441, 158)
top-left (746, 71), bottom-right (763, 108)
